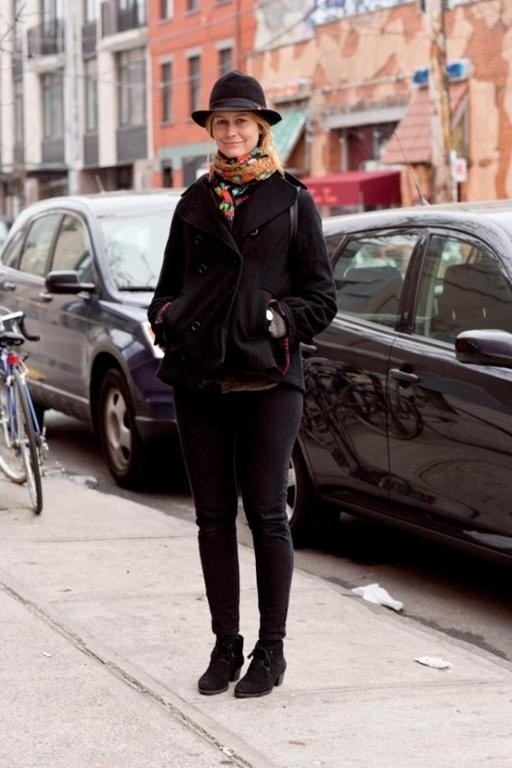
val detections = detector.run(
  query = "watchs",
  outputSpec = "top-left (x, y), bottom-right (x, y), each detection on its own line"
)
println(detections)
top-left (266, 306), bottom-right (275, 332)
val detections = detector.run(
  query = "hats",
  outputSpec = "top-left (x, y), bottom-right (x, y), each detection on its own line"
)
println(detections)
top-left (189, 69), bottom-right (283, 130)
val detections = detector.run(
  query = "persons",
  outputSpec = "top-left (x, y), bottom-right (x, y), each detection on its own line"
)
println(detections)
top-left (146, 69), bottom-right (338, 699)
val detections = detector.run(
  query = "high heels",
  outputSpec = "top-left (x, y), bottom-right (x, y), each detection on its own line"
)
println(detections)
top-left (197, 633), bottom-right (244, 696)
top-left (233, 639), bottom-right (287, 698)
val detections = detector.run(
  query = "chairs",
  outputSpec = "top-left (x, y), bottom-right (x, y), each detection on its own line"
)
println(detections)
top-left (342, 260), bottom-right (511, 343)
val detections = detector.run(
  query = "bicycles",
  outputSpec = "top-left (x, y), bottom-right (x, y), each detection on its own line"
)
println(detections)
top-left (0, 283), bottom-right (49, 514)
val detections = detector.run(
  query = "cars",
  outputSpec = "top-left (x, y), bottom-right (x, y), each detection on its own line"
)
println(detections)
top-left (285, 199), bottom-right (512, 560)
top-left (1, 187), bottom-right (187, 491)
